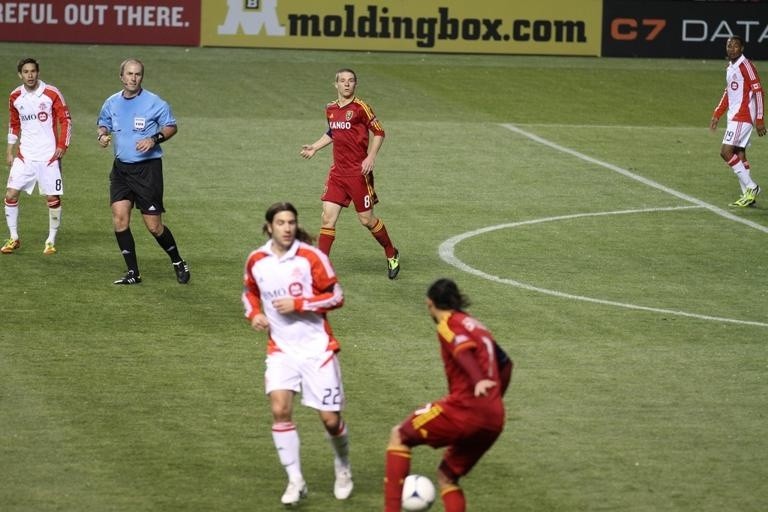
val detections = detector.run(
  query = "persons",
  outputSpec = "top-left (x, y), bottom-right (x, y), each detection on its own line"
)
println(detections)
top-left (96, 56), bottom-right (190, 284)
top-left (300, 68), bottom-right (401, 278)
top-left (382, 278), bottom-right (513, 511)
top-left (1, 57), bottom-right (71, 256)
top-left (710, 35), bottom-right (767, 207)
top-left (242, 202), bottom-right (355, 507)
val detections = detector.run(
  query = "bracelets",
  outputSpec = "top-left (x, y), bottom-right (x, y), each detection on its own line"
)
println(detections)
top-left (97, 133), bottom-right (107, 140)
top-left (151, 132), bottom-right (164, 144)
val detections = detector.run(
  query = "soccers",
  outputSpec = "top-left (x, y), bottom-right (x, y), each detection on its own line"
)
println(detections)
top-left (400, 474), bottom-right (435, 511)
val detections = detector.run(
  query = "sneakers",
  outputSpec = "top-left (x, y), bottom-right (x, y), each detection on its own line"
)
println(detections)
top-left (43, 242), bottom-right (57, 255)
top-left (333, 454), bottom-right (354, 500)
top-left (280, 479), bottom-right (307, 505)
top-left (172, 260), bottom-right (190, 284)
top-left (386, 247), bottom-right (400, 279)
top-left (738, 184), bottom-right (762, 207)
top-left (113, 270), bottom-right (142, 285)
top-left (728, 194), bottom-right (757, 208)
top-left (1, 237), bottom-right (20, 253)
